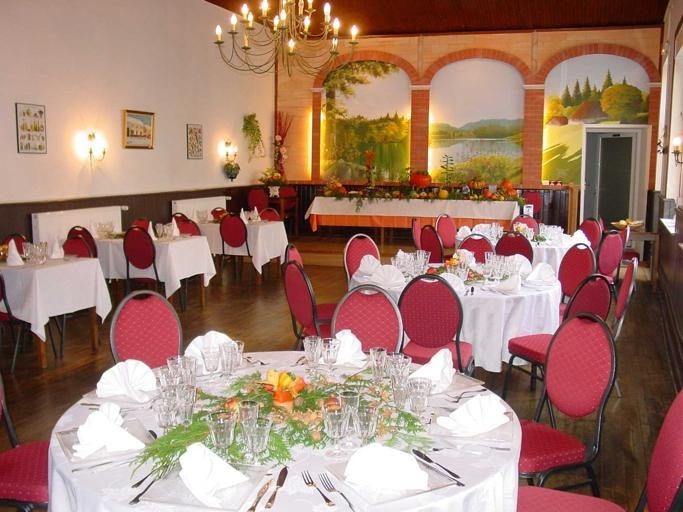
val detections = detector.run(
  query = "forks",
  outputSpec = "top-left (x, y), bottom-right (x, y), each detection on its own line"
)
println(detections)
top-left (128, 455), bottom-right (176, 505)
top-left (444, 387), bottom-right (486, 402)
top-left (317, 473), bottom-right (356, 511)
top-left (301, 470), bottom-right (333, 506)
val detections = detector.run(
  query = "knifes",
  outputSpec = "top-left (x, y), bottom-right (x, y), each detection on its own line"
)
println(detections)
top-left (266, 466), bottom-right (287, 508)
top-left (247, 477), bottom-right (272, 510)
top-left (411, 449), bottom-right (459, 478)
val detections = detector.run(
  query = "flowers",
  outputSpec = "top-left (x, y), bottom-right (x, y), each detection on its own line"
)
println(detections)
top-left (257, 167), bottom-right (284, 186)
top-left (361, 150), bottom-right (376, 184)
top-left (272, 110), bottom-right (295, 174)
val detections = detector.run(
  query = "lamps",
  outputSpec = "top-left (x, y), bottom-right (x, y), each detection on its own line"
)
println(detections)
top-left (670, 135), bottom-right (683, 167)
top-left (218, 139), bottom-right (239, 163)
top-left (654, 138), bottom-right (668, 157)
top-left (69, 126), bottom-right (110, 168)
top-left (211, 0), bottom-right (359, 82)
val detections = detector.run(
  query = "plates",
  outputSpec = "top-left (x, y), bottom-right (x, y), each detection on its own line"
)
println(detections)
top-left (81, 377), bottom-right (161, 405)
top-left (441, 370), bottom-right (487, 392)
top-left (54, 415), bottom-right (155, 465)
top-left (324, 458), bottom-right (456, 506)
top-left (426, 405), bottom-right (516, 446)
top-left (134, 464), bottom-right (268, 511)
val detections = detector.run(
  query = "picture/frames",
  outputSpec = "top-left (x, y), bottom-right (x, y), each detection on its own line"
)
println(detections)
top-left (184, 123), bottom-right (205, 161)
top-left (120, 109), bottom-right (158, 151)
top-left (13, 102), bottom-right (48, 158)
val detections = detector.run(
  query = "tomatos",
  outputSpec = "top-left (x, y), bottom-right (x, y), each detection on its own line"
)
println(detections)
top-left (410, 172), bottom-right (432, 188)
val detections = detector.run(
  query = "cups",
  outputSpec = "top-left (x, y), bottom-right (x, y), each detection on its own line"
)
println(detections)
top-left (20, 241), bottom-right (47, 265)
top-left (154, 224), bottom-right (174, 240)
top-left (477, 222), bottom-right (564, 243)
top-left (390, 249), bottom-right (522, 281)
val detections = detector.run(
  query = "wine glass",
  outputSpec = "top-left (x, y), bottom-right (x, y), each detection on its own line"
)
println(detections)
top-left (150, 335), bottom-right (432, 462)
